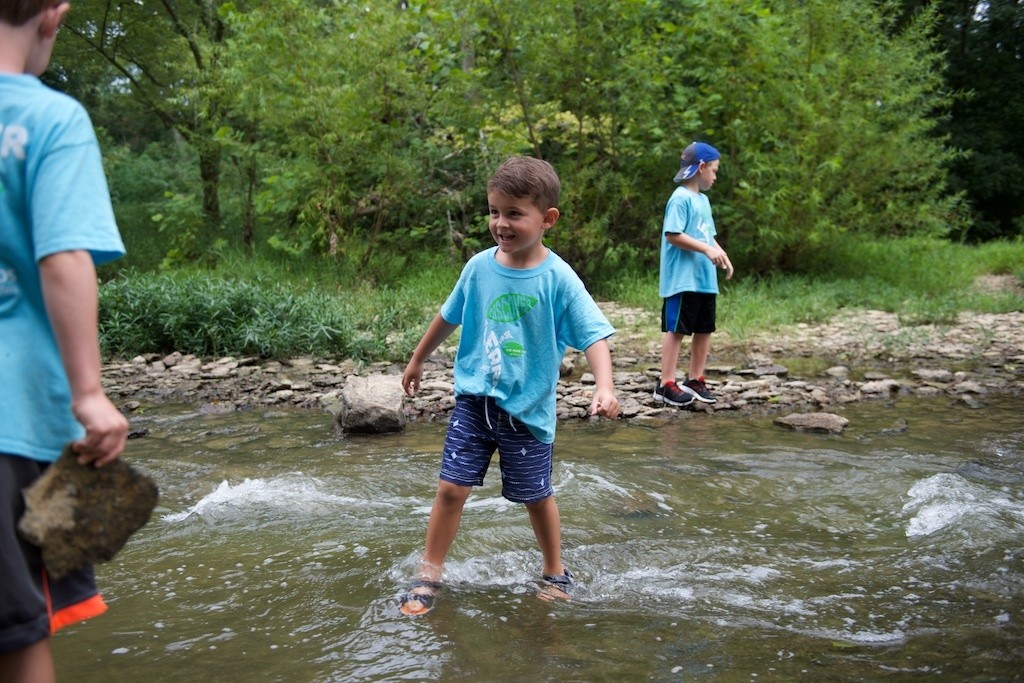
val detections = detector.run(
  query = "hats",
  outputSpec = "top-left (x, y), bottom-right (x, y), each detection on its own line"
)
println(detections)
top-left (673, 141), bottom-right (721, 183)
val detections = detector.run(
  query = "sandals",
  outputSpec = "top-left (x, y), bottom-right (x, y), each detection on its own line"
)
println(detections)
top-left (400, 581), bottom-right (443, 615)
top-left (543, 568), bottom-right (574, 594)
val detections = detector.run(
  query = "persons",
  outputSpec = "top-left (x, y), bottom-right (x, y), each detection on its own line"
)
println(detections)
top-left (401, 156), bottom-right (620, 586)
top-left (0, 0), bottom-right (129, 683)
top-left (653, 142), bottom-right (734, 405)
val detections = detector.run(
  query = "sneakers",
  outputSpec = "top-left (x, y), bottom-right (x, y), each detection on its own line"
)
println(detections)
top-left (678, 378), bottom-right (717, 403)
top-left (653, 380), bottom-right (696, 406)
